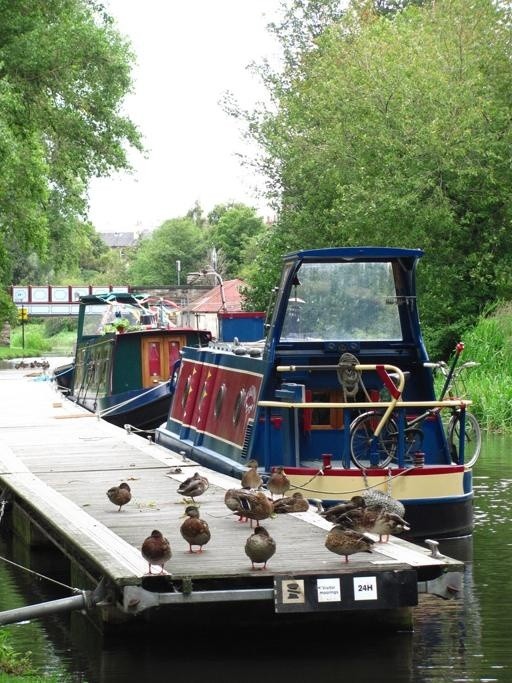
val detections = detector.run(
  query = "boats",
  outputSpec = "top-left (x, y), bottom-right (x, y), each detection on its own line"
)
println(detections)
top-left (153, 243), bottom-right (475, 544)
top-left (67, 290), bottom-right (215, 430)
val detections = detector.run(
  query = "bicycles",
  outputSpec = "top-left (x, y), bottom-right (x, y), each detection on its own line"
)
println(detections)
top-left (346, 359), bottom-right (484, 470)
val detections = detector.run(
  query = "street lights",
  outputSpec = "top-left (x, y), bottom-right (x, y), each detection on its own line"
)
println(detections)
top-left (175, 259), bottom-right (180, 285)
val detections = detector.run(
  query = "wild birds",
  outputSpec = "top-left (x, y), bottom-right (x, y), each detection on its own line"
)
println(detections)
top-left (173, 470), bottom-right (209, 504)
top-left (223, 457), bottom-right (309, 526)
top-left (105, 481), bottom-right (131, 514)
top-left (244, 525), bottom-right (277, 570)
top-left (316, 493), bottom-right (414, 566)
top-left (176, 505), bottom-right (212, 553)
top-left (140, 529), bottom-right (174, 575)
